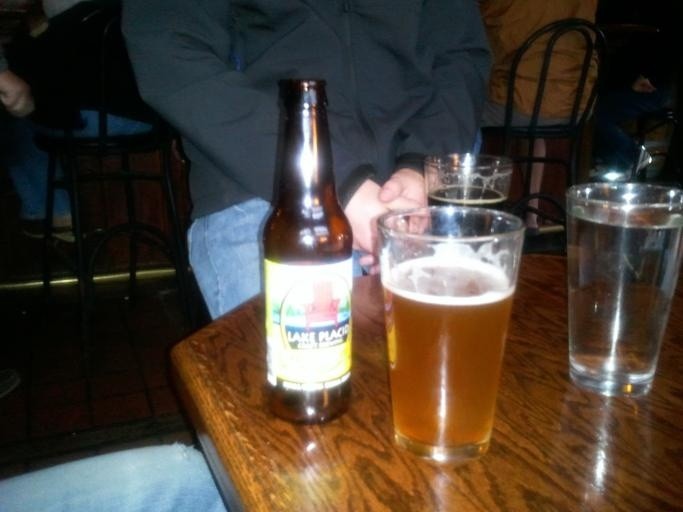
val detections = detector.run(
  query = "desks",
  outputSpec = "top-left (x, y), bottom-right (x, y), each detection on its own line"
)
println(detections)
top-left (171, 251), bottom-right (682, 512)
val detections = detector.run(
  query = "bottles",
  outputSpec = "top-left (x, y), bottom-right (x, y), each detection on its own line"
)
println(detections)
top-left (256, 78), bottom-right (354, 425)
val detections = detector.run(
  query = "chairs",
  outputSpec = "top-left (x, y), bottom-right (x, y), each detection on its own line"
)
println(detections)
top-left (484, 18), bottom-right (609, 249)
top-left (36, 1), bottom-right (202, 440)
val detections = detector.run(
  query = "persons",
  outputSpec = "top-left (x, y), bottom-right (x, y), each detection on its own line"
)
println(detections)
top-left (2, 1), bottom-right (173, 243)
top-left (0, 438), bottom-right (229, 511)
top-left (476, 0), bottom-right (683, 135)
top-left (121, 0), bottom-right (495, 323)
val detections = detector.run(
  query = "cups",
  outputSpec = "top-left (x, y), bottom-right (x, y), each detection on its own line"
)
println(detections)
top-left (421, 151), bottom-right (515, 267)
top-left (564, 181), bottom-right (683, 398)
top-left (375, 205), bottom-right (527, 464)
